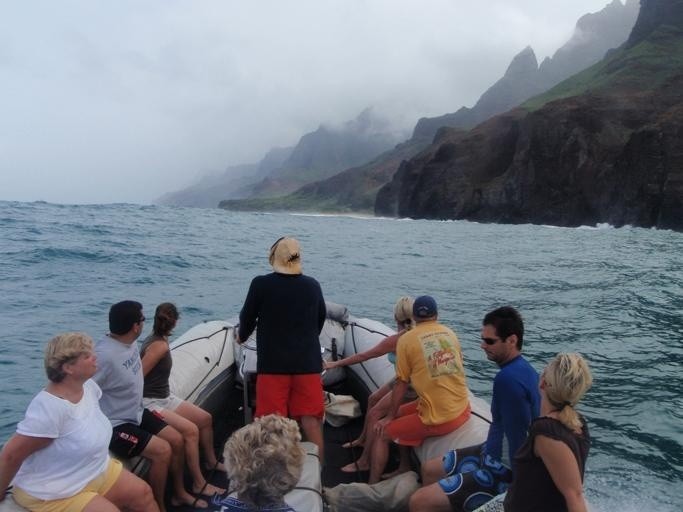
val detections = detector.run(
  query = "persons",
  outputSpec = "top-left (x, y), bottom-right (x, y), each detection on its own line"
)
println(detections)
top-left (365, 294), bottom-right (470, 485)
top-left (0, 332), bottom-right (163, 511)
top-left (232, 235), bottom-right (326, 468)
top-left (87, 300), bottom-right (208, 511)
top-left (469, 350), bottom-right (594, 510)
top-left (211, 413), bottom-right (307, 511)
top-left (406, 304), bottom-right (543, 511)
top-left (138, 302), bottom-right (228, 498)
top-left (321, 295), bottom-right (417, 476)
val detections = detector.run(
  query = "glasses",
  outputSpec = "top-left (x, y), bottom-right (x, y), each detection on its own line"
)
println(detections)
top-left (480, 337), bottom-right (504, 346)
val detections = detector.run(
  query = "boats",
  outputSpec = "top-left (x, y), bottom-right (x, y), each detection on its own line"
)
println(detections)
top-left (0, 303), bottom-right (496, 512)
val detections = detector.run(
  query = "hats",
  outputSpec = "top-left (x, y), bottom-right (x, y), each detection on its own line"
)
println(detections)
top-left (270, 237), bottom-right (304, 276)
top-left (411, 295), bottom-right (439, 318)
